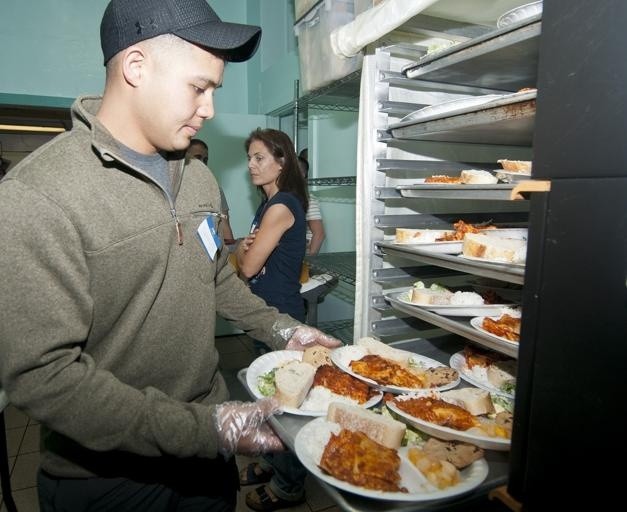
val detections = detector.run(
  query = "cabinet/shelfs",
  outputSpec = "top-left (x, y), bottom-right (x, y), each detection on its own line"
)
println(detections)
top-left (354, 10), bottom-right (541, 511)
top-left (292, 71), bottom-right (359, 336)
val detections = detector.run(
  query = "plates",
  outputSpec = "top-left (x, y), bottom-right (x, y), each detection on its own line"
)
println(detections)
top-left (396, 287), bottom-right (521, 318)
top-left (298, 415), bottom-right (488, 506)
top-left (328, 341), bottom-right (462, 393)
top-left (392, 226), bottom-right (528, 269)
top-left (497, 0), bottom-right (541, 28)
top-left (448, 348), bottom-right (514, 399)
top-left (399, 88), bottom-right (536, 124)
top-left (246, 350), bottom-right (383, 418)
top-left (466, 315), bottom-right (520, 350)
top-left (386, 392), bottom-right (511, 453)
top-left (491, 160), bottom-right (530, 186)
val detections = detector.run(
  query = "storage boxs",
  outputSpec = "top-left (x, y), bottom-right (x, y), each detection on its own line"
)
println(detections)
top-left (292, 0), bottom-right (363, 98)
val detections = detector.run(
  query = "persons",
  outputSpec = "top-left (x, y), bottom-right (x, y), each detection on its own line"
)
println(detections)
top-left (297, 156), bottom-right (326, 254)
top-left (300, 149), bottom-right (308, 162)
top-left (236, 127), bottom-right (309, 511)
top-left (0, 0), bottom-right (343, 512)
top-left (187, 139), bottom-right (234, 241)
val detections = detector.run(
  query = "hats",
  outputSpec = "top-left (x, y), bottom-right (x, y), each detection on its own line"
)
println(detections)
top-left (100, 0), bottom-right (262, 66)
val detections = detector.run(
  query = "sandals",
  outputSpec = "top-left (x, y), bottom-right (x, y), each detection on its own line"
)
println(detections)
top-left (239, 463), bottom-right (276, 485)
top-left (246, 485), bottom-right (306, 512)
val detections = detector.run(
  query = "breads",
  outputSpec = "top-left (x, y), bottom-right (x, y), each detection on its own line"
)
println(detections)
top-left (410, 288), bottom-right (456, 305)
top-left (303, 343), bottom-right (334, 368)
top-left (423, 174), bottom-right (462, 184)
top-left (464, 342), bottom-right (503, 370)
top-left (461, 167), bottom-right (498, 186)
top-left (397, 396), bottom-right (472, 430)
top-left (461, 232), bottom-right (528, 263)
top-left (488, 359), bottom-right (518, 389)
top-left (320, 429), bottom-right (401, 492)
top-left (483, 315), bottom-right (522, 342)
top-left (394, 227), bottom-right (454, 243)
top-left (497, 158), bottom-right (532, 174)
top-left (444, 386), bottom-right (497, 419)
top-left (349, 354), bottom-right (423, 390)
top-left (324, 404), bottom-right (407, 452)
top-left (274, 360), bottom-right (315, 408)
top-left (358, 336), bottom-right (412, 366)
